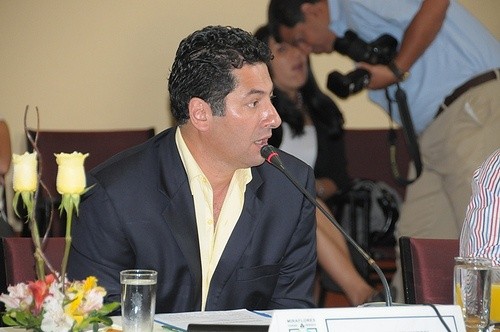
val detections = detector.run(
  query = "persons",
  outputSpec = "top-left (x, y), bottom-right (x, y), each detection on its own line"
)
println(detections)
top-left (255, 23), bottom-right (399, 307)
top-left (460, 147), bottom-right (499, 285)
top-left (268, 0), bottom-right (500, 305)
top-left (65, 26), bottom-right (318, 318)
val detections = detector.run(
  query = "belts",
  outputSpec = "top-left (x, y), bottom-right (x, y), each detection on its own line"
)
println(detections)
top-left (436, 69), bottom-right (500, 116)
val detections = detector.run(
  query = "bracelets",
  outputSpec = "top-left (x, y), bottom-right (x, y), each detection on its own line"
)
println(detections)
top-left (387, 63), bottom-right (409, 81)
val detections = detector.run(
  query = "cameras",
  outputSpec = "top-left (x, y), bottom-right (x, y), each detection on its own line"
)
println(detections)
top-left (327, 30), bottom-right (398, 98)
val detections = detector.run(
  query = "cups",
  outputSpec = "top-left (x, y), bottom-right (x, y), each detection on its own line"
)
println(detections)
top-left (453, 256), bottom-right (500, 332)
top-left (119, 269), bottom-right (158, 332)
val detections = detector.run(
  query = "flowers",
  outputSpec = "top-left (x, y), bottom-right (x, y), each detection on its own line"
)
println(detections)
top-left (0, 150), bottom-right (121, 332)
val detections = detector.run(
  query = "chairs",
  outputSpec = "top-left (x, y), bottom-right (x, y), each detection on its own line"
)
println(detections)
top-left (0, 129), bottom-right (459, 305)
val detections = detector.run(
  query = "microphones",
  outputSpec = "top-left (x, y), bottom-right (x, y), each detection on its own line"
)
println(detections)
top-left (260, 145), bottom-right (393, 306)
